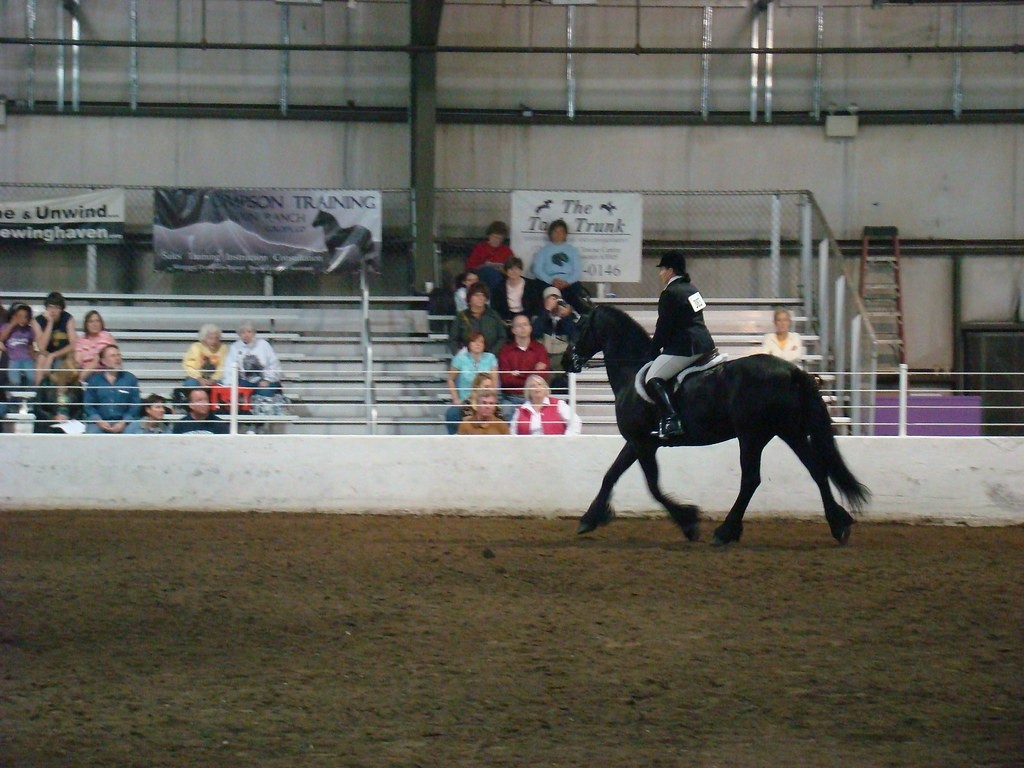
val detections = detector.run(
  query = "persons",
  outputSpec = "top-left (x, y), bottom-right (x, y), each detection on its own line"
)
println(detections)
top-left (764, 307), bottom-right (808, 370)
top-left (0, 289), bottom-right (174, 436)
top-left (645, 253), bottom-right (719, 439)
top-left (444, 218), bottom-right (590, 435)
top-left (173, 319), bottom-right (288, 438)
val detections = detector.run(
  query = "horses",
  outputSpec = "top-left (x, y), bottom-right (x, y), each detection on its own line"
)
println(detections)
top-left (313, 210), bottom-right (376, 266)
top-left (569, 297), bottom-right (869, 546)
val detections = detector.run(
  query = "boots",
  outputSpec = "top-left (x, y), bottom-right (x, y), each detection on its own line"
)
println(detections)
top-left (645, 377), bottom-right (682, 435)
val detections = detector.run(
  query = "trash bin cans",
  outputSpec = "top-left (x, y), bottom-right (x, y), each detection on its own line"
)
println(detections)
top-left (3, 391), bottom-right (37, 432)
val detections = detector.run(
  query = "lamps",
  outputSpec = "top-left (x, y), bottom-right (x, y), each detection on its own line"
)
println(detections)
top-left (826, 101), bottom-right (860, 137)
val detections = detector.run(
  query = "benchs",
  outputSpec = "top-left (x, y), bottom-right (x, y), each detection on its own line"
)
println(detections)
top-left (0, 293), bottom-right (854, 437)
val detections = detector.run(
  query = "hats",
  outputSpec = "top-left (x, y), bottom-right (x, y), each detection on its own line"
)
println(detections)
top-left (544, 287), bottom-right (562, 299)
top-left (44, 291), bottom-right (66, 309)
top-left (655, 250), bottom-right (686, 270)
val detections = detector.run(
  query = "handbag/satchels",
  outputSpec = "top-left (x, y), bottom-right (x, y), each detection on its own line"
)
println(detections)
top-left (211, 385), bottom-right (250, 415)
top-left (249, 393), bottom-right (292, 416)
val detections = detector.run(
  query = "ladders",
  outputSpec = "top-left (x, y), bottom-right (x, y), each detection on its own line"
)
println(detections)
top-left (861, 226), bottom-right (910, 389)
